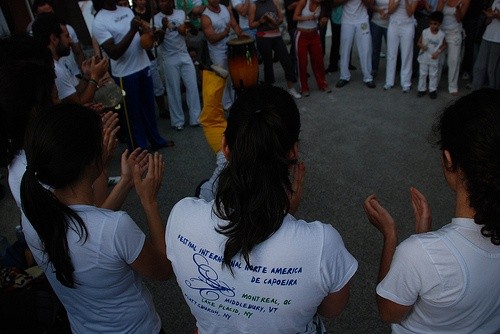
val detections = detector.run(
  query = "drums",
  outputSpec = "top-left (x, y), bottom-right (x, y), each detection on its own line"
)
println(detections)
top-left (225, 36), bottom-right (261, 93)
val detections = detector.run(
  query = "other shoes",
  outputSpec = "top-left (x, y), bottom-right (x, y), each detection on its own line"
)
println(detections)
top-left (322, 86), bottom-right (331, 93)
top-left (380, 51), bottom-right (385, 57)
top-left (466, 82), bottom-right (473, 88)
top-left (176, 126), bottom-right (183, 129)
top-left (156, 140), bottom-right (174, 147)
top-left (336, 79), bottom-right (349, 87)
top-left (301, 91), bottom-right (310, 95)
top-left (366, 80), bottom-right (377, 88)
top-left (328, 64), bottom-right (340, 72)
top-left (349, 63), bottom-right (357, 71)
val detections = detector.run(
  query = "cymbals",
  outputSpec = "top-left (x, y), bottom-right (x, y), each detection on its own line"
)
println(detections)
top-left (94, 82), bottom-right (126, 110)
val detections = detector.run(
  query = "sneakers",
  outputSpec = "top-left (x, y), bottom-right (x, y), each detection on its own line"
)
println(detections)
top-left (402, 86), bottom-right (410, 93)
top-left (448, 86), bottom-right (458, 94)
top-left (430, 90), bottom-right (437, 99)
top-left (416, 90), bottom-right (426, 98)
top-left (384, 83), bottom-right (392, 89)
top-left (285, 87), bottom-right (301, 98)
top-left (106, 175), bottom-right (121, 184)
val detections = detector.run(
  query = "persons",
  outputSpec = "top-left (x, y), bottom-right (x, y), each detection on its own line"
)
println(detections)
top-left (28, 0), bottom-right (84, 79)
top-left (285, 0), bottom-right (332, 96)
top-left (371, 0), bottom-right (470, 95)
top-left (364, 88), bottom-right (500, 334)
top-left (20, 103), bottom-right (171, 334)
top-left (188, 47), bottom-right (209, 103)
top-left (116, 0), bottom-right (157, 61)
top-left (466, 0), bottom-right (500, 89)
top-left (164, 85), bottom-right (358, 334)
top-left (417, 11), bottom-right (447, 98)
top-left (325, 0), bottom-right (375, 88)
top-left (175, 0), bottom-right (247, 74)
top-left (149, 0), bottom-right (202, 130)
top-left (231, 0), bottom-right (301, 98)
top-left (0, 33), bottom-right (121, 211)
top-left (92, 0), bottom-right (174, 155)
top-left (32, 13), bottom-right (108, 104)
top-left (194, 61), bottom-right (230, 201)
